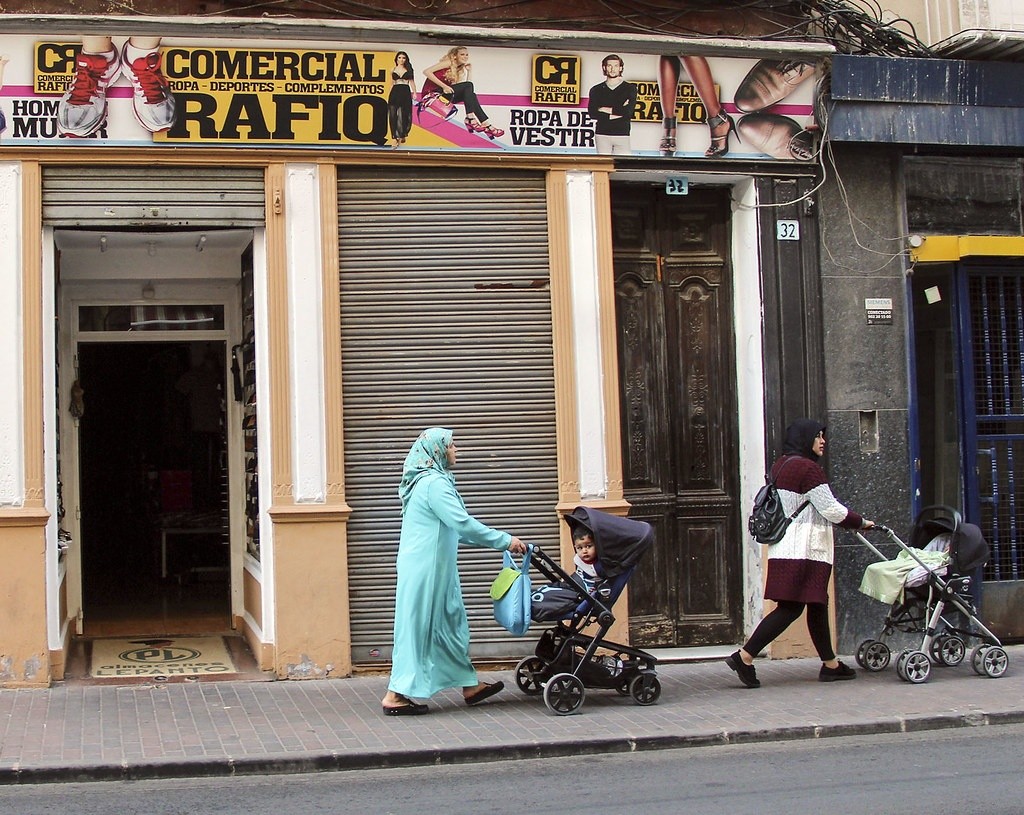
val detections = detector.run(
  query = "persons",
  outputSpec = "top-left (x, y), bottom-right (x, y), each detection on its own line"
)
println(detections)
top-left (415, 47), bottom-right (504, 140)
top-left (532, 524), bottom-right (603, 620)
top-left (586, 53), bottom-right (639, 154)
top-left (381, 422), bottom-right (532, 715)
top-left (656, 54), bottom-right (742, 159)
top-left (723, 418), bottom-right (877, 688)
top-left (55, 35), bottom-right (179, 139)
top-left (387, 51), bottom-right (419, 149)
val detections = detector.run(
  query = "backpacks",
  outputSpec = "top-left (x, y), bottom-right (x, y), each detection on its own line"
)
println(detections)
top-left (749, 457), bottom-right (810, 545)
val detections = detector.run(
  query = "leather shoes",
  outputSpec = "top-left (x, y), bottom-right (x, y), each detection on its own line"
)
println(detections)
top-left (734, 59), bottom-right (815, 113)
top-left (736, 113), bottom-right (812, 160)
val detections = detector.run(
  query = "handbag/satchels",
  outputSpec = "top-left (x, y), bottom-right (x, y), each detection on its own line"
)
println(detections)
top-left (490, 543), bottom-right (534, 636)
top-left (418, 88), bottom-right (457, 128)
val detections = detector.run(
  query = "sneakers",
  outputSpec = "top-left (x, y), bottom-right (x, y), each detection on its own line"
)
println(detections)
top-left (120, 39), bottom-right (177, 132)
top-left (819, 662), bottom-right (856, 681)
top-left (726, 651), bottom-right (760, 687)
top-left (58, 42), bottom-right (121, 139)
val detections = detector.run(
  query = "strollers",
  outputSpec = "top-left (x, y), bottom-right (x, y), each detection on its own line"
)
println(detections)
top-left (850, 505), bottom-right (1009, 684)
top-left (514, 505), bottom-right (663, 717)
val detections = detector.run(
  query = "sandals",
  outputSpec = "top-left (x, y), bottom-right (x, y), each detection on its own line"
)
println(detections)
top-left (383, 699), bottom-right (429, 716)
top-left (465, 681), bottom-right (504, 704)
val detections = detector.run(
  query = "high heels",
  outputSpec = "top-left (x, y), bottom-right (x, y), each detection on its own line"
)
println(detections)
top-left (464, 117), bottom-right (487, 133)
top-left (483, 125), bottom-right (505, 140)
top-left (705, 108), bottom-right (742, 158)
top-left (660, 116), bottom-right (678, 156)
